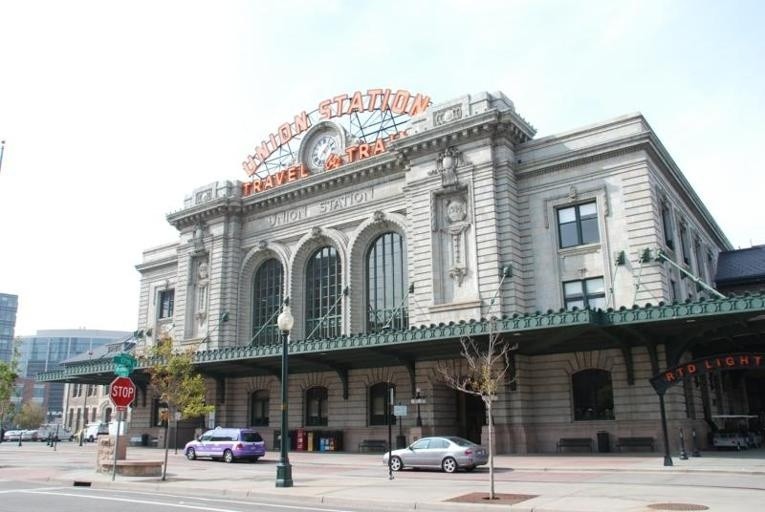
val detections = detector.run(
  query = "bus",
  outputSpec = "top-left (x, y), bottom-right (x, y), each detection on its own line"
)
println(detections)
top-left (710, 412), bottom-right (762, 452)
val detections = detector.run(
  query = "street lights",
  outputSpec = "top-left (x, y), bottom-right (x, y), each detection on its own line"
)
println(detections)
top-left (275, 301), bottom-right (295, 490)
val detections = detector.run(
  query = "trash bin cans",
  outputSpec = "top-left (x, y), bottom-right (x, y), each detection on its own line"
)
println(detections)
top-left (597, 430), bottom-right (610, 453)
top-left (142, 434), bottom-right (149, 446)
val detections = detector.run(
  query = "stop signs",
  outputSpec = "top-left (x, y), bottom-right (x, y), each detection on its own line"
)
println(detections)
top-left (109, 377), bottom-right (136, 410)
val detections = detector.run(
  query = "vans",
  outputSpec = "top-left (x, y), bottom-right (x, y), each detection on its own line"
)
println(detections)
top-left (37, 424), bottom-right (74, 442)
top-left (83, 422), bottom-right (109, 442)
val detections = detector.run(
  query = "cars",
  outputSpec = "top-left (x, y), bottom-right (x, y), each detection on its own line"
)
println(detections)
top-left (5, 428), bottom-right (37, 442)
top-left (382, 435), bottom-right (488, 474)
top-left (183, 426), bottom-right (266, 463)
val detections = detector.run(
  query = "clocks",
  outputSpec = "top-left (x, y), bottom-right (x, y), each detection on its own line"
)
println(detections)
top-left (311, 136), bottom-right (337, 169)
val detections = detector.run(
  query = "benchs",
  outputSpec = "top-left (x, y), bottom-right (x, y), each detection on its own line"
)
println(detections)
top-left (150, 437), bottom-right (159, 446)
top-left (128, 437), bottom-right (142, 446)
top-left (616, 437), bottom-right (655, 453)
top-left (556, 437), bottom-right (593, 453)
top-left (359, 439), bottom-right (387, 453)
